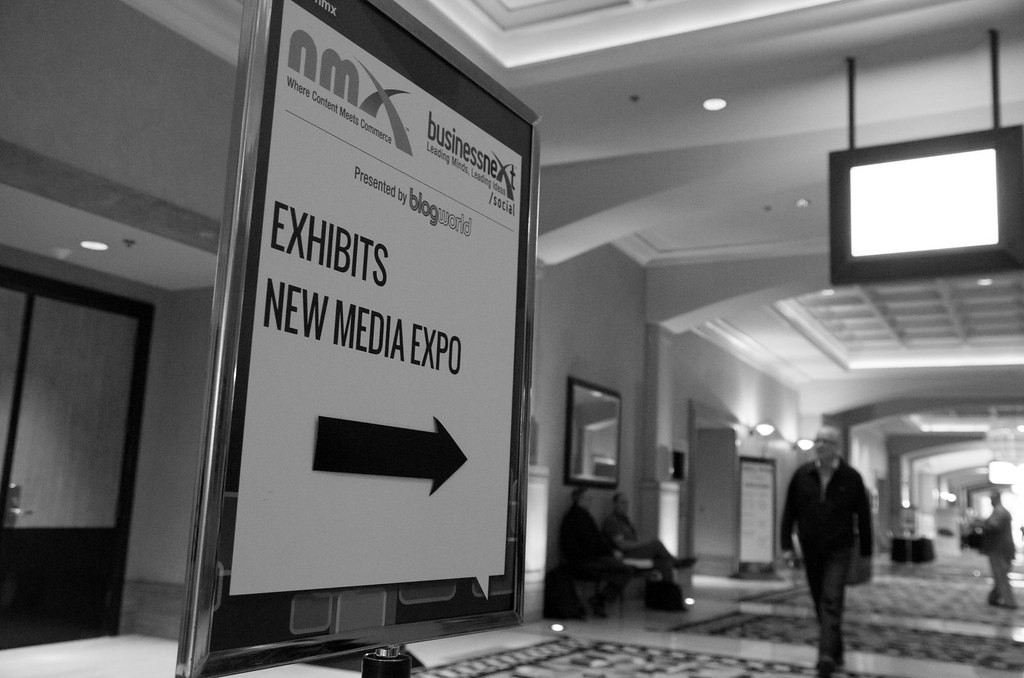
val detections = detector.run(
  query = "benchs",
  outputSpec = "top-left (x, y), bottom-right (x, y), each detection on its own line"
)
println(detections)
top-left (557, 520), bottom-right (658, 608)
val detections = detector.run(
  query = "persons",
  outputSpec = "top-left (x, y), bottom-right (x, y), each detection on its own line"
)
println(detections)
top-left (602, 491), bottom-right (698, 613)
top-left (557, 486), bottom-right (636, 619)
top-left (779, 426), bottom-right (874, 678)
top-left (968, 489), bottom-right (1019, 610)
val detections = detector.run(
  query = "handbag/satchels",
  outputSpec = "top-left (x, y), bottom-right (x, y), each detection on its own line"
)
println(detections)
top-left (644, 580), bottom-right (688, 613)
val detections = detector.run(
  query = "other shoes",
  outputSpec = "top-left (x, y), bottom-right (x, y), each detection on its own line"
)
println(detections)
top-left (817, 654), bottom-right (843, 676)
top-left (590, 592), bottom-right (607, 619)
top-left (674, 558), bottom-right (697, 569)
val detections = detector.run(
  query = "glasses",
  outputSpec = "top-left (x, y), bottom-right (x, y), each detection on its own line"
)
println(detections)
top-left (815, 438), bottom-right (840, 447)
